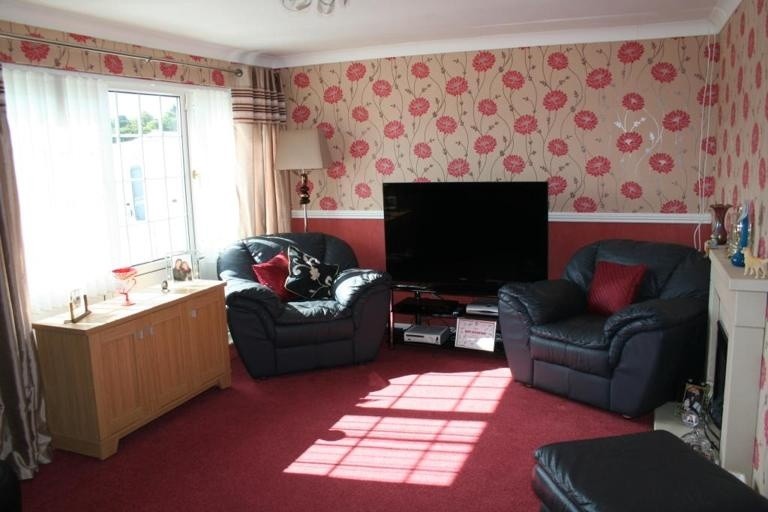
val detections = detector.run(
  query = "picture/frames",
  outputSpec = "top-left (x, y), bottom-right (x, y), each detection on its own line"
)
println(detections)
top-left (164, 248), bottom-right (200, 292)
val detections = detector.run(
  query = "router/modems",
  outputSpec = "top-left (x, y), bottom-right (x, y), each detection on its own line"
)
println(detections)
top-left (466, 302), bottom-right (498, 316)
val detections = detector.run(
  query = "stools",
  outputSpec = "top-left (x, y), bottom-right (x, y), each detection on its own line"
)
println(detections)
top-left (531, 426), bottom-right (768, 512)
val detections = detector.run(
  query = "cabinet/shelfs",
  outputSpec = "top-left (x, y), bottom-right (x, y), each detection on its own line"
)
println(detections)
top-left (28, 297), bottom-right (188, 462)
top-left (182, 284), bottom-right (232, 401)
top-left (392, 285), bottom-right (502, 352)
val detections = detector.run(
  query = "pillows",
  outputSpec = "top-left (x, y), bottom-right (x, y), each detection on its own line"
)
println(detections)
top-left (250, 251), bottom-right (300, 300)
top-left (585, 260), bottom-right (647, 317)
top-left (596, 251), bottom-right (683, 302)
top-left (243, 235), bottom-right (304, 265)
top-left (285, 245), bottom-right (340, 303)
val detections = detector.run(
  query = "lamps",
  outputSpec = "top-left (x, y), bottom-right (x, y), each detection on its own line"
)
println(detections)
top-left (280, 0), bottom-right (351, 18)
top-left (275, 129), bottom-right (334, 232)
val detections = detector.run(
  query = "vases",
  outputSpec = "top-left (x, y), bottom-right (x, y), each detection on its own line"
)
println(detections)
top-left (709, 203), bottom-right (735, 245)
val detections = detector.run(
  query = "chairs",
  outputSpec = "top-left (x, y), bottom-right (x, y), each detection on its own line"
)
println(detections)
top-left (216, 232), bottom-right (393, 380)
top-left (495, 237), bottom-right (711, 419)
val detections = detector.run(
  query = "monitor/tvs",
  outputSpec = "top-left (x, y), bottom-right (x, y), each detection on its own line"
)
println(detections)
top-left (382, 181), bottom-right (548, 296)
